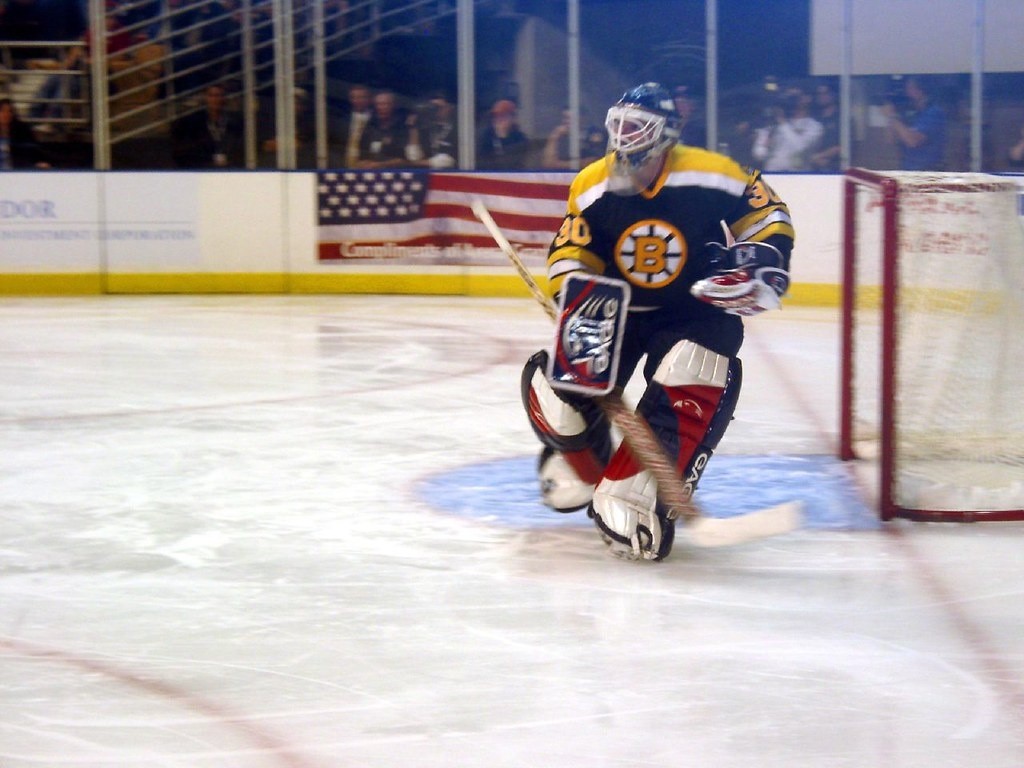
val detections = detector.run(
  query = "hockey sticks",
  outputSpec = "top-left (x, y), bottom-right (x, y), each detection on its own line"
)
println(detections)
top-left (468, 196), bottom-right (805, 548)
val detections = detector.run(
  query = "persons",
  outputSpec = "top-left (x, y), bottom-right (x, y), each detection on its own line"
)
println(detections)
top-left (522, 82), bottom-right (794, 561)
top-left (0, 0), bottom-right (1024, 175)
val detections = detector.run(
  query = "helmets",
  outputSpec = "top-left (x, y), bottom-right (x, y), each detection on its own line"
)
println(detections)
top-left (606, 80), bottom-right (680, 168)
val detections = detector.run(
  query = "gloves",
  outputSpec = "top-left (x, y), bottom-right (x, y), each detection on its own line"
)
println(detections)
top-left (690, 239), bottom-right (790, 315)
